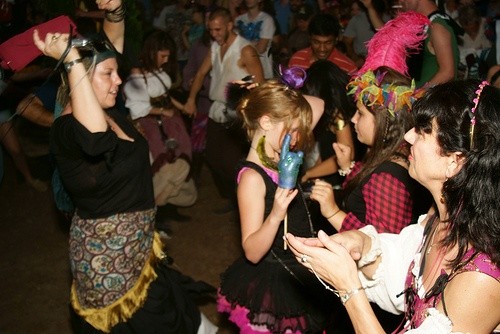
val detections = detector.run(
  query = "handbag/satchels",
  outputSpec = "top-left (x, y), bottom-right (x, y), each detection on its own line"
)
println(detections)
top-left (167, 177), bottom-right (198, 206)
top-left (149, 157), bottom-right (190, 207)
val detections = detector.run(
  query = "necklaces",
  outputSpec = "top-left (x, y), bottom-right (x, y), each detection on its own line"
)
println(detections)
top-left (426, 222), bottom-right (444, 256)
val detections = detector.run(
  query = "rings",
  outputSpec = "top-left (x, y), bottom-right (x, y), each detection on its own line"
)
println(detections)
top-left (302, 256), bottom-right (306, 262)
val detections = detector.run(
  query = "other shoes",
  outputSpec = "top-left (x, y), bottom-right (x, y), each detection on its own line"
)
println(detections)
top-left (171, 212), bottom-right (189, 220)
top-left (212, 198), bottom-right (231, 212)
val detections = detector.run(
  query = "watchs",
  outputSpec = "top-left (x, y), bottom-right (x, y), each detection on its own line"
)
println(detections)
top-left (63, 59), bottom-right (83, 71)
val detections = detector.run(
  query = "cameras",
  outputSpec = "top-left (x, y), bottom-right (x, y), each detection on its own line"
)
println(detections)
top-left (71, 39), bottom-right (89, 47)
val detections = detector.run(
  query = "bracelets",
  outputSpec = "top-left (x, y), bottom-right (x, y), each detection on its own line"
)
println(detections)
top-left (101, 8), bottom-right (127, 23)
top-left (159, 107), bottom-right (164, 114)
top-left (335, 285), bottom-right (363, 304)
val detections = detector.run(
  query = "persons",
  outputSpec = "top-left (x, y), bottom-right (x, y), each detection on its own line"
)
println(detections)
top-left (389, 0), bottom-right (460, 88)
top-left (124, 33), bottom-right (198, 235)
top-left (286, 13), bottom-right (358, 80)
top-left (0, 0), bottom-right (500, 222)
top-left (181, 9), bottom-right (266, 215)
top-left (32, 0), bottom-right (219, 334)
top-left (215, 75), bottom-right (347, 334)
top-left (283, 83), bottom-right (500, 334)
